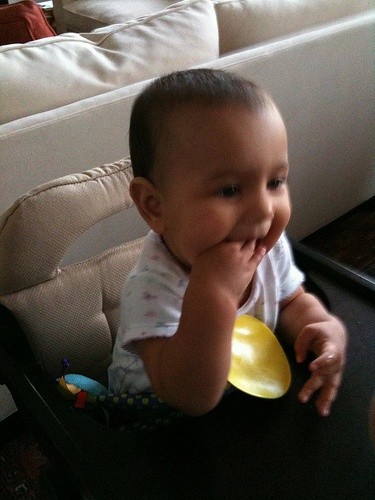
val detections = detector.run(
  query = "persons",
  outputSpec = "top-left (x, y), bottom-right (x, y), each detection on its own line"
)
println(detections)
top-left (107, 69), bottom-right (350, 416)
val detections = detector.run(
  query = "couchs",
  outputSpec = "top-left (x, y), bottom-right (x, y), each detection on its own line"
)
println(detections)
top-left (0, 0), bottom-right (375, 268)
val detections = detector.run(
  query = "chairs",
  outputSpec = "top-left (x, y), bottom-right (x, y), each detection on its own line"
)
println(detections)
top-left (0, 155), bottom-right (375, 500)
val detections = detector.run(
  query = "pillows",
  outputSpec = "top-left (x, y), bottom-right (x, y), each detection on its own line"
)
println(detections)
top-left (0, 0), bottom-right (219, 124)
